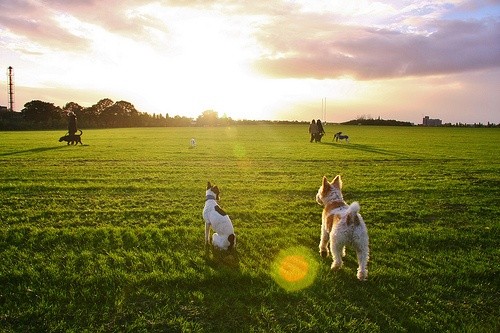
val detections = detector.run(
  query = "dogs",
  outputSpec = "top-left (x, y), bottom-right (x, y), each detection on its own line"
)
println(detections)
top-left (201, 180), bottom-right (237, 257)
top-left (59, 129), bottom-right (83, 146)
top-left (331, 131), bottom-right (348, 142)
top-left (190, 136), bottom-right (196, 148)
top-left (309, 133), bottom-right (324, 143)
top-left (315, 174), bottom-right (372, 282)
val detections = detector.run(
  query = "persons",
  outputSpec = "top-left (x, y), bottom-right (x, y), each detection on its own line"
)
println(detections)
top-left (316, 119), bottom-right (326, 143)
top-left (308, 119), bottom-right (319, 143)
top-left (67, 111), bottom-right (77, 146)
